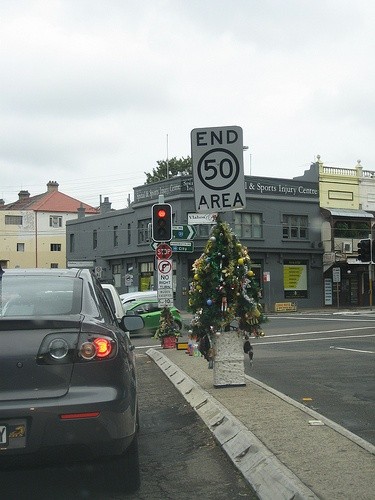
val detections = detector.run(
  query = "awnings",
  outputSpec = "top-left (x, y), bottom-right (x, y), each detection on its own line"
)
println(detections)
top-left (320, 207), bottom-right (374, 218)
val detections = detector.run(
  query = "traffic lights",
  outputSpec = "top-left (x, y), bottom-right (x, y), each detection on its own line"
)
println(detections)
top-left (152, 203), bottom-right (173, 243)
top-left (357, 239), bottom-right (373, 263)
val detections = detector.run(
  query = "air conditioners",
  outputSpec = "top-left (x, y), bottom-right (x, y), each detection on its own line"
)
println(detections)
top-left (343, 242), bottom-right (352, 253)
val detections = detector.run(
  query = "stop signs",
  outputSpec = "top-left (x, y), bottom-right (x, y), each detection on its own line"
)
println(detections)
top-left (155, 244), bottom-right (172, 260)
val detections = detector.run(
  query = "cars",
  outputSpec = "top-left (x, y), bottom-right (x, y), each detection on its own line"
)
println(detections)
top-left (0, 268), bottom-right (149, 495)
top-left (119, 291), bottom-right (157, 313)
top-left (1, 283), bottom-right (131, 339)
top-left (123, 300), bottom-right (182, 336)
